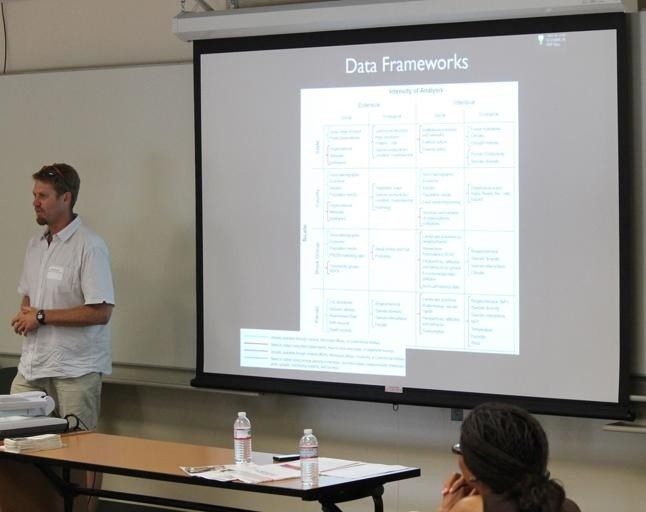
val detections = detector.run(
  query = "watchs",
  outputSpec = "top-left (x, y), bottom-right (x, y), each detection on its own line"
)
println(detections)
top-left (35, 310), bottom-right (47, 325)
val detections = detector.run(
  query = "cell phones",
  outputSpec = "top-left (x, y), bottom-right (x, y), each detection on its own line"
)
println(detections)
top-left (273, 454), bottom-right (300, 461)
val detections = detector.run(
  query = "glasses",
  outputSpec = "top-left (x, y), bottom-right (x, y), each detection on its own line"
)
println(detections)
top-left (40, 165), bottom-right (72, 190)
top-left (451, 442), bottom-right (464, 456)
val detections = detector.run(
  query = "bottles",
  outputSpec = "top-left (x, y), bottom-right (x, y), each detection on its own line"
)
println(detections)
top-left (234, 411), bottom-right (251, 465)
top-left (299, 429), bottom-right (319, 490)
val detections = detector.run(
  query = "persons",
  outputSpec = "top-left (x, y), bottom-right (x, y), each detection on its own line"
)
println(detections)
top-left (6, 159), bottom-right (118, 510)
top-left (430, 401), bottom-right (583, 512)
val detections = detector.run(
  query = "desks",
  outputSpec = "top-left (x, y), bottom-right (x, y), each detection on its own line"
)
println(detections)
top-left (1, 432), bottom-right (422, 511)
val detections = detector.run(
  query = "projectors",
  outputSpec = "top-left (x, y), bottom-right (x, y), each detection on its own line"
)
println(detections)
top-left (0, 391), bottom-right (68, 440)
